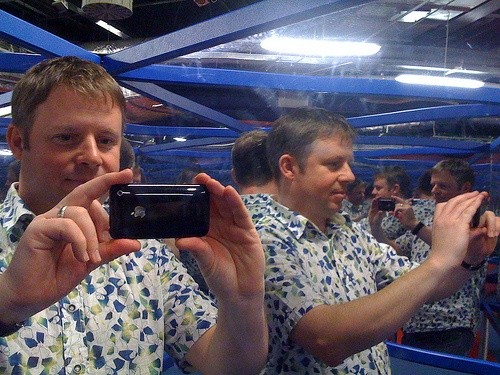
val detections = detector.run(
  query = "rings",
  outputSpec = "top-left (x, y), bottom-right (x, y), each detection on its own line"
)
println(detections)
top-left (401, 203), bottom-right (403, 206)
top-left (57, 205), bottom-right (68, 218)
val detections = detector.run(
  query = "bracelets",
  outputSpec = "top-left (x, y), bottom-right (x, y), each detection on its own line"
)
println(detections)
top-left (412, 221), bottom-right (424, 235)
top-left (461, 260), bottom-right (487, 271)
top-left (0, 321), bottom-right (24, 337)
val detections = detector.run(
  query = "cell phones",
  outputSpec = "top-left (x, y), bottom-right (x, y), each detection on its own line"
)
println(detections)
top-left (472, 206), bottom-right (480, 227)
top-left (376, 198), bottom-right (395, 211)
top-left (109, 184), bottom-right (210, 239)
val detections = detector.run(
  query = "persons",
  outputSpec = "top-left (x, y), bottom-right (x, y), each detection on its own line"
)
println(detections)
top-left (103, 129), bottom-right (488, 375)
top-left (0, 160), bottom-right (20, 205)
top-left (255, 106), bottom-right (500, 375)
top-left (0, 58), bottom-right (270, 375)
